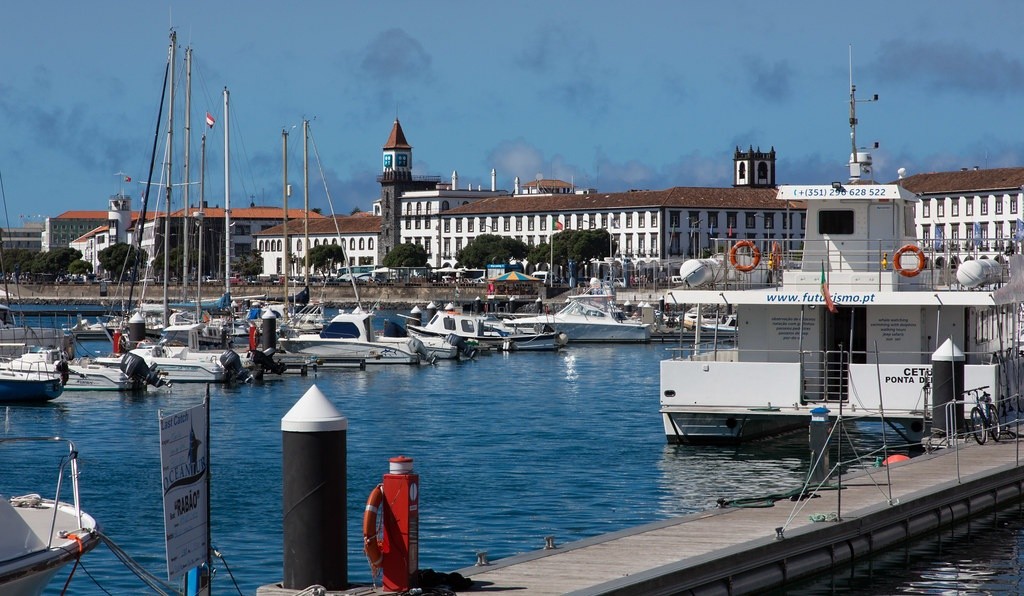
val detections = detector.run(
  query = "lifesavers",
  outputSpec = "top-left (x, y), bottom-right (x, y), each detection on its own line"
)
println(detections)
top-left (730, 240), bottom-right (761, 272)
top-left (892, 244), bottom-right (926, 278)
top-left (772, 241), bottom-right (781, 270)
top-left (363, 483), bottom-right (385, 570)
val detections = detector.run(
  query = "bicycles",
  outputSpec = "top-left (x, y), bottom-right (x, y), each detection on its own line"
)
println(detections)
top-left (960, 384), bottom-right (1001, 445)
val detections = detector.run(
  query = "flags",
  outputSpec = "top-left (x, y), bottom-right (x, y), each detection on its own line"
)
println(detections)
top-left (552, 218), bottom-right (563, 230)
top-left (820, 261), bottom-right (840, 313)
top-left (709, 219), bottom-right (713, 236)
top-left (1016, 218), bottom-right (1024, 241)
top-left (934, 225), bottom-right (944, 251)
top-left (729, 219), bottom-right (732, 236)
top-left (671, 219), bottom-right (675, 237)
top-left (375, 303), bottom-right (383, 310)
top-left (975, 224), bottom-right (982, 246)
top-left (124, 175), bottom-right (131, 182)
top-left (206, 111), bottom-right (215, 129)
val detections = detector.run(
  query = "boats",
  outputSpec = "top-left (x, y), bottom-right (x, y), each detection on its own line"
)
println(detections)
top-left (0, 434), bottom-right (106, 596)
top-left (654, 44), bottom-right (1024, 449)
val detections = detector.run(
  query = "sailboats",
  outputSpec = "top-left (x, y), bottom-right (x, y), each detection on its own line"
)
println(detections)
top-left (1, 4), bottom-right (741, 404)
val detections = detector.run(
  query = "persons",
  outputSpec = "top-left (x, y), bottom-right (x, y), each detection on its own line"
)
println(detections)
top-left (629, 274), bottom-right (649, 288)
top-left (440, 277), bottom-right (477, 287)
top-left (766, 241), bottom-right (781, 287)
